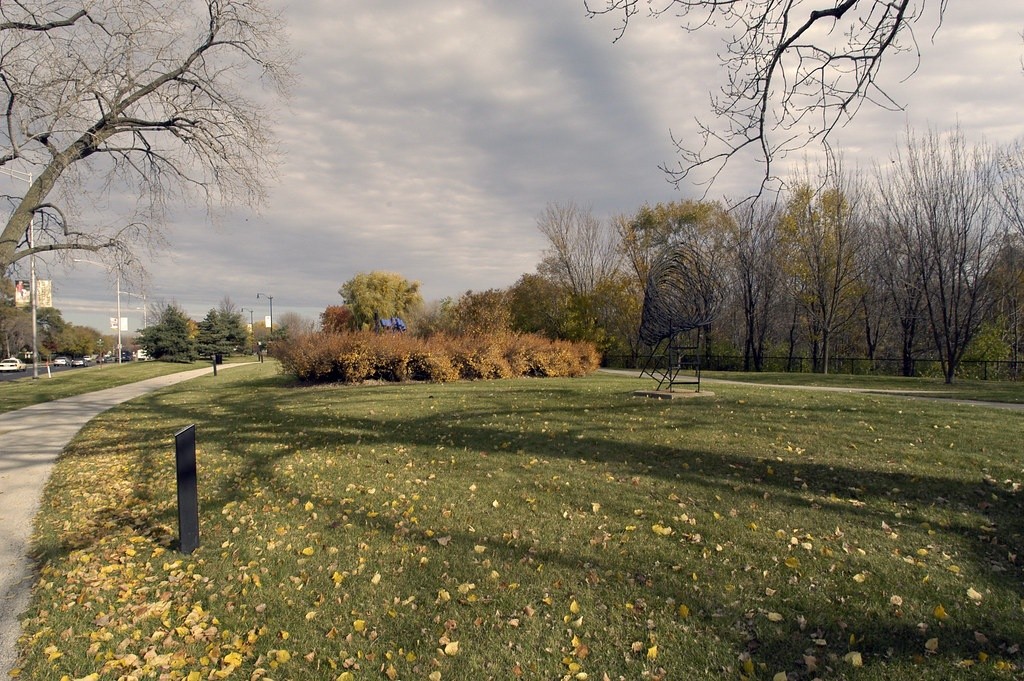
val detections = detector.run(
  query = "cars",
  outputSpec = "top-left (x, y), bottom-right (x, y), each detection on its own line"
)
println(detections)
top-left (53, 349), bottom-right (151, 367)
top-left (0, 356), bottom-right (27, 372)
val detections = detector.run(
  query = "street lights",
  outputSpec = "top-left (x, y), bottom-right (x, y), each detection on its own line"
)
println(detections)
top-left (116, 290), bottom-right (147, 330)
top-left (241, 306), bottom-right (254, 334)
top-left (256, 292), bottom-right (274, 336)
top-left (72, 258), bottom-right (122, 364)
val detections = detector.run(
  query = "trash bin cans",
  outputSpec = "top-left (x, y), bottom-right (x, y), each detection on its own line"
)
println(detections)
top-left (216, 355), bottom-right (222, 364)
